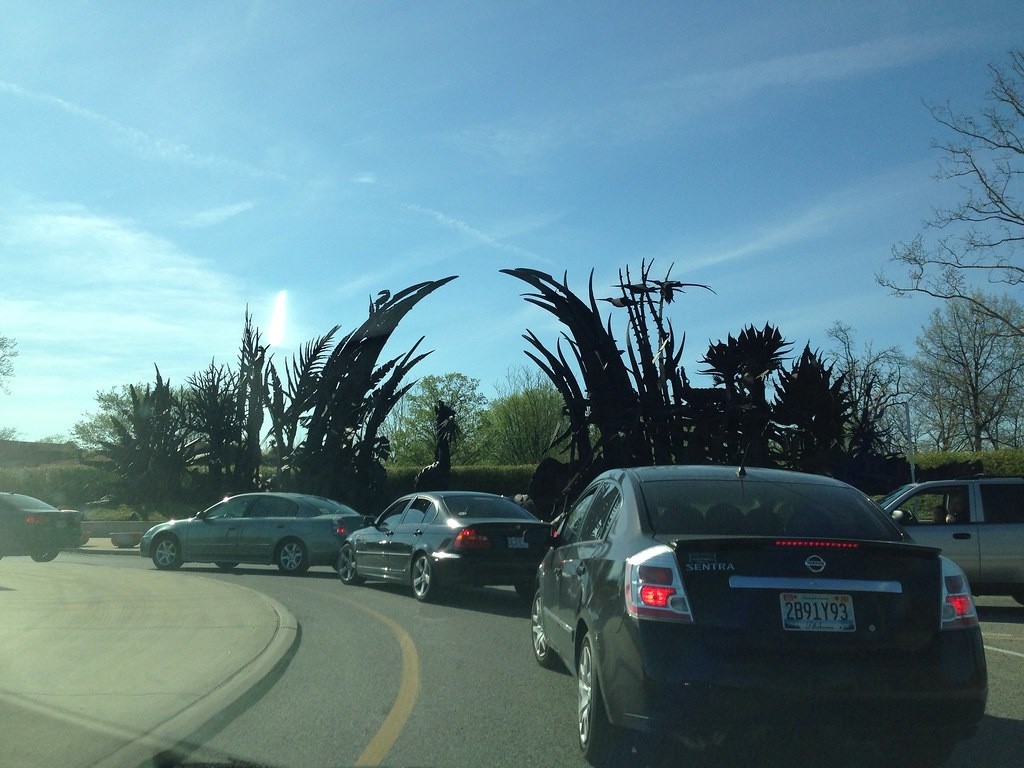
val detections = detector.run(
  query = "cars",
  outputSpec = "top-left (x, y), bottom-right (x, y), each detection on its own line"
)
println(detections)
top-left (522, 466), bottom-right (990, 768)
top-left (138, 491), bottom-right (378, 576)
top-left (332, 491), bottom-right (552, 605)
top-left (0, 491), bottom-right (82, 563)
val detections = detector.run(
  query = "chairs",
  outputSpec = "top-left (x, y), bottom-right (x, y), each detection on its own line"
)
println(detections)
top-left (447, 500), bottom-right (473, 518)
top-left (256, 501), bottom-right (277, 516)
top-left (950, 497), bottom-right (971, 523)
top-left (661, 503), bottom-right (838, 537)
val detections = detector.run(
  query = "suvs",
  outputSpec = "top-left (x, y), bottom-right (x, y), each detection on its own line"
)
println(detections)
top-left (872, 477), bottom-right (1024, 607)
